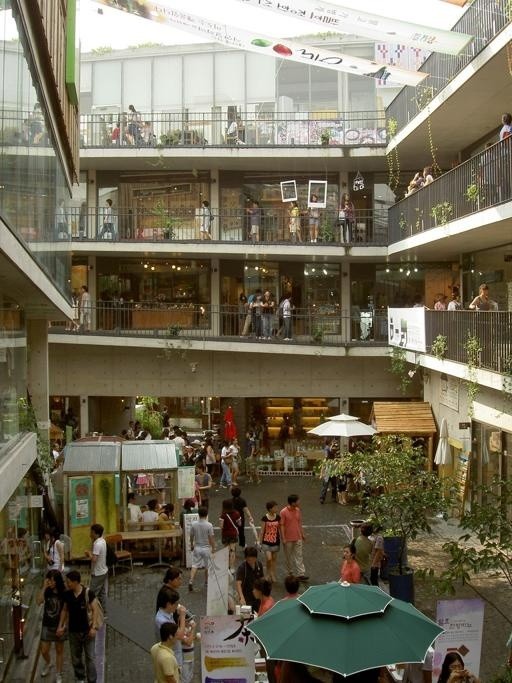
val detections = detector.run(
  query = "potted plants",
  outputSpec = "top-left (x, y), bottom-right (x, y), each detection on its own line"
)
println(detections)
top-left (151, 200), bottom-right (183, 239)
top-left (363, 471), bottom-right (460, 604)
top-left (317, 125), bottom-right (335, 145)
top-left (157, 321), bottom-right (192, 361)
top-left (313, 435), bottom-right (431, 580)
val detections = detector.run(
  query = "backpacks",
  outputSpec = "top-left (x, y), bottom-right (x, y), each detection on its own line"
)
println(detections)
top-left (224, 452), bottom-right (233, 464)
top-left (105, 542), bottom-right (118, 567)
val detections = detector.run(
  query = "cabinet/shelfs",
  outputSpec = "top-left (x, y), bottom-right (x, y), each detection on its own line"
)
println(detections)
top-left (266, 397), bottom-right (330, 438)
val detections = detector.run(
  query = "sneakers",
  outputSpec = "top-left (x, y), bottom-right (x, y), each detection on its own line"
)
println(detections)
top-left (41, 662), bottom-right (52, 675)
top-left (55, 672), bottom-right (64, 683)
top-left (311, 239), bottom-right (314, 243)
top-left (296, 575), bottom-right (309, 580)
top-left (314, 239), bottom-right (317, 243)
top-left (77, 680), bottom-right (85, 683)
top-left (188, 581), bottom-right (194, 591)
top-left (204, 584), bottom-right (207, 590)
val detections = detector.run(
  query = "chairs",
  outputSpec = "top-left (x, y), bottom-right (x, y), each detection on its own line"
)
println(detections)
top-left (224, 128), bottom-right (237, 144)
top-left (104, 534), bottom-right (133, 577)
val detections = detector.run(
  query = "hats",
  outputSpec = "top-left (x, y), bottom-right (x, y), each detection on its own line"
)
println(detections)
top-left (185, 444), bottom-right (194, 448)
top-left (437, 294), bottom-right (448, 301)
top-left (191, 439), bottom-right (202, 446)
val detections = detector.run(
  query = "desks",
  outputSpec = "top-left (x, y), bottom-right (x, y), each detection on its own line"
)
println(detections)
top-left (120, 529), bottom-right (183, 569)
top-left (184, 130), bottom-right (196, 144)
top-left (238, 126), bottom-right (245, 142)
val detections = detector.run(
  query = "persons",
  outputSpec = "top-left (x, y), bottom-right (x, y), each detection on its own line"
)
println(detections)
top-left (3, 103), bottom-right (511, 683)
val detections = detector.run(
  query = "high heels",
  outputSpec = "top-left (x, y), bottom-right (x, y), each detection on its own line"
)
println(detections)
top-left (266, 574), bottom-right (272, 583)
top-left (271, 575), bottom-right (277, 583)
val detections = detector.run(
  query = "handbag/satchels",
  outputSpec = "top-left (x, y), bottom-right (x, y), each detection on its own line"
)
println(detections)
top-left (236, 527), bottom-right (239, 535)
top-left (255, 541), bottom-right (263, 553)
top-left (210, 214), bottom-right (214, 221)
top-left (85, 588), bottom-right (104, 630)
